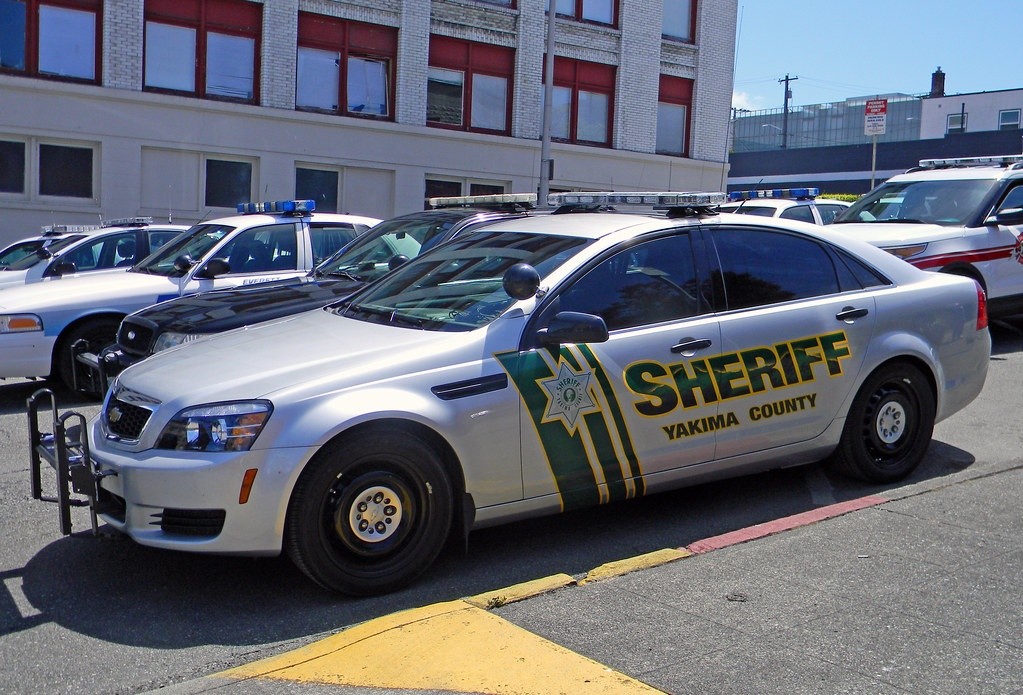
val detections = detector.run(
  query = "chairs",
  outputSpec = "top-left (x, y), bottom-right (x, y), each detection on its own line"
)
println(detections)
top-left (114, 241), bottom-right (135, 267)
top-left (555, 267), bottom-right (635, 328)
top-left (242, 240), bottom-right (273, 274)
top-left (268, 231), bottom-right (297, 270)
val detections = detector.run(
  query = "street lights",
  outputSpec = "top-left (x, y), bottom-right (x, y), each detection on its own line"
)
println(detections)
top-left (762, 124), bottom-right (787, 149)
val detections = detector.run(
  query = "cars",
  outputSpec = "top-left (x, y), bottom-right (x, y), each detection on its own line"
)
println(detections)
top-left (27, 191), bottom-right (991, 602)
top-left (0, 224), bottom-right (134, 271)
top-left (0, 200), bottom-right (457, 404)
top-left (851, 197), bottom-right (937, 220)
top-left (820, 154), bottom-right (1023, 321)
top-left (709, 188), bottom-right (877, 225)
top-left (0, 216), bottom-right (253, 291)
top-left (71, 193), bottom-right (559, 402)
